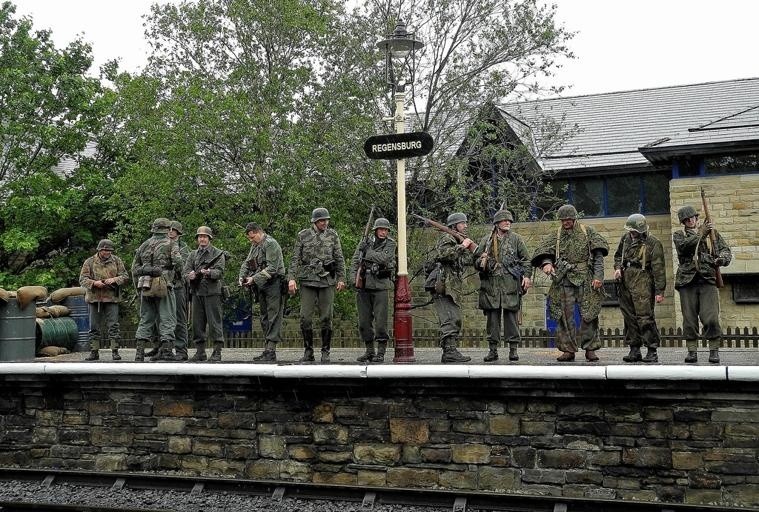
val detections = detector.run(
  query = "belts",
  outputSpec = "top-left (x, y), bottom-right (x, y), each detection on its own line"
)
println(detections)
top-left (623, 261), bottom-right (651, 270)
top-left (685, 255), bottom-right (701, 262)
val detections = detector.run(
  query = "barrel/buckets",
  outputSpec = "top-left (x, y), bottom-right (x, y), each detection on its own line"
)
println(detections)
top-left (545, 292), bottom-right (579, 348)
top-left (0, 295), bottom-right (91, 361)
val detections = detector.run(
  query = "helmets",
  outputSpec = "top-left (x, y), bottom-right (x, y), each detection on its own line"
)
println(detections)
top-left (446, 213), bottom-right (467, 227)
top-left (493, 210), bottom-right (514, 225)
top-left (623, 213), bottom-right (649, 234)
top-left (195, 226), bottom-right (212, 240)
top-left (557, 205), bottom-right (578, 220)
top-left (678, 205), bottom-right (699, 225)
top-left (371, 218), bottom-right (391, 232)
top-left (96, 239), bottom-right (115, 252)
top-left (310, 208), bottom-right (330, 223)
top-left (150, 218), bottom-right (173, 234)
top-left (171, 220), bottom-right (182, 235)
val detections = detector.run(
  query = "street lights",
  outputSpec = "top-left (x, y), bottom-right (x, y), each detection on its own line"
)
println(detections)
top-left (371, 18), bottom-right (424, 363)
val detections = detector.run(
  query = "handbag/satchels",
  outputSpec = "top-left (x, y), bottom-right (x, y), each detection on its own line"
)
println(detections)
top-left (142, 277), bottom-right (168, 298)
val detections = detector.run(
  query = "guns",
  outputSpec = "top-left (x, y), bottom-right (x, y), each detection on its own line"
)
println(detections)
top-left (356, 207), bottom-right (374, 289)
top-left (700, 187), bottom-right (724, 288)
top-left (188, 250), bottom-right (224, 296)
top-left (412, 213), bottom-right (479, 254)
top-left (480, 201), bottom-right (505, 271)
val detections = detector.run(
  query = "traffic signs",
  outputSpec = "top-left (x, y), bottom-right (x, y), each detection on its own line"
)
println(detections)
top-left (361, 132), bottom-right (434, 162)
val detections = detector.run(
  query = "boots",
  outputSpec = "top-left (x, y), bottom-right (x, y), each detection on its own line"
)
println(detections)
top-left (110, 340), bottom-right (121, 360)
top-left (253, 340), bottom-right (276, 361)
top-left (134, 336), bottom-right (223, 363)
top-left (623, 346), bottom-right (642, 362)
top-left (440, 337), bottom-right (519, 363)
top-left (374, 341), bottom-right (387, 362)
top-left (85, 339), bottom-right (99, 360)
top-left (299, 329), bottom-right (315, 361)
top-left (321, 328), bottom-right (333, 361)
top-left (357, 340), bottom-right (375, 361)
top-left (709, 339), bottom-right (720, 362)
top-left (685, 340), bottom-right (697, 362)
top-left (643, 347), bottom-right (658, 362)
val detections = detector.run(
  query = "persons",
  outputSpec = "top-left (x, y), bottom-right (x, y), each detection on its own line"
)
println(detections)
top-left (531, 205), bottom-right (608, 363)
top-left (144, 221), bottom-right (193, 361)
top-left (130, 217), bottom-right (184, 362)
top-left (287, 207), bottom-right (346, 361)
top-left (613, 214), bottom-right (667, 363)
top-left (473, 210), bottom-right (533, 362)
top-left (238, 222), bottom-right (286, 361)
top-left (674, 208), bottom-right (731, 363)
top-left (78, 239), bottom-right (129, 361)
top-left (350, 218), bottom-right (396, 363)
top-left (424, 212), bottom-right (474, 363)
top-left (183, 226), bottom-right (225, 363)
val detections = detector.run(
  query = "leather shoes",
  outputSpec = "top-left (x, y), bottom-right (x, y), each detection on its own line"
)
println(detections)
top-left (557, 352), bottom-right (575, 361)
top-left (585, 350), bottom-right (599, 360)
